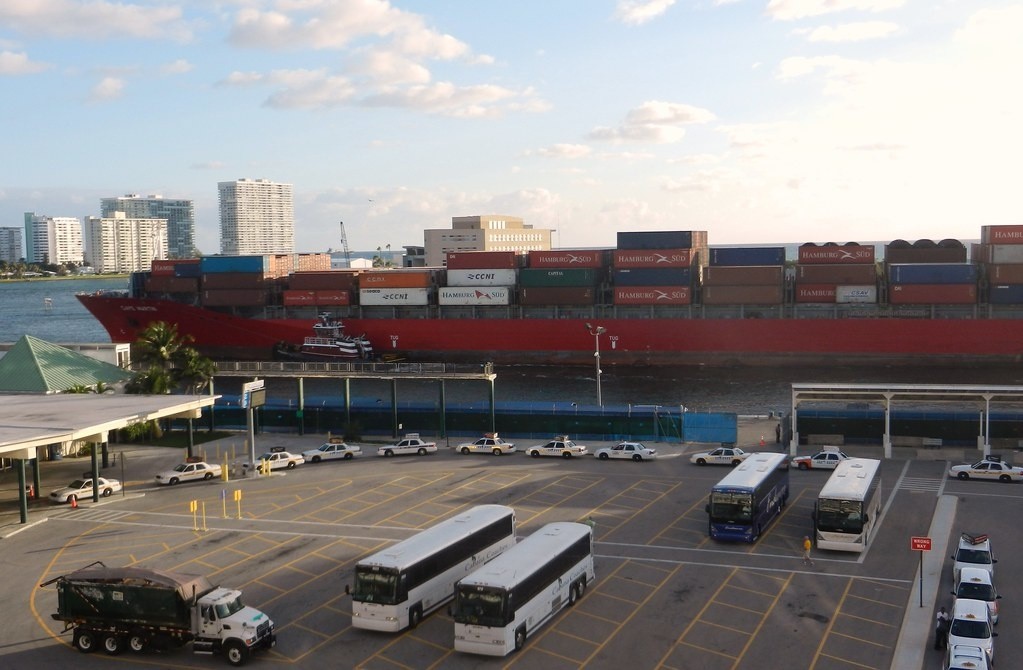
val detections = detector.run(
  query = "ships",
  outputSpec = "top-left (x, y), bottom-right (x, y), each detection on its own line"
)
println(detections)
top-left (73, 201), bottom-right (1023, 370)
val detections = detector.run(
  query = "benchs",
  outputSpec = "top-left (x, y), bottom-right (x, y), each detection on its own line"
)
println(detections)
top-left (922, 438), bottom-right (943, 448)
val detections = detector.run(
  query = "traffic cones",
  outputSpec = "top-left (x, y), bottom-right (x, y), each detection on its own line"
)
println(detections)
top-left (760, 435), bottom-right (766, 446)
top-left (71, 495), bottom-right (77, 508)
top-left (30, 485), bottom-right (34, 496)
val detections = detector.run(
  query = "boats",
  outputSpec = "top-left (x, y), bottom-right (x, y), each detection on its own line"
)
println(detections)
top-left (272, 312), bottom-right (382, 363)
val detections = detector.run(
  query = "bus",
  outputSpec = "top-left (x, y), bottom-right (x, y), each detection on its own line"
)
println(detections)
top-left (344, 504), bottom-right (517, 634)
top-left (704, 452), bottom-right (790, 544)
top-left (446, 521), bottom-right (597, 659)
top-left (812, 457), bottom-right (884, 554)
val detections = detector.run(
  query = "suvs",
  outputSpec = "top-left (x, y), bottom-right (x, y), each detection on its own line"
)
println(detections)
top-left (943, 533), bottom-right (1002, 670)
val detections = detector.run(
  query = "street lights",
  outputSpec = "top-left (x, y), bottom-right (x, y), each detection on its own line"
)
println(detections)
top-left (585, 322), bottom-right (608, 405)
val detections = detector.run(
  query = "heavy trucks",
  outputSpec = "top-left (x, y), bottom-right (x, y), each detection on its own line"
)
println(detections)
top-left (51, 567), bottom-right (278, 666)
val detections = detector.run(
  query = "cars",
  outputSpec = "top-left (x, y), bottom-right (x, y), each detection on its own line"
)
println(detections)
top-left (690, 444), bottom-right (751, 467)
top-left (377, 433), bottom-right (437, 457)
top-left (241, 446), bottom-right (305, 474)
top-left (301, 438), bottom-right (363, 463)
top-left (455, 432), bottom-right (517, 456)
top-left (525, 435), bottom-right (589, 459)
top-left (948, 455), bottom-right (1023, 482)
top-left (593, 440), bottom-right (657, 462)
top-left (48, 472), bottom-right (123, 504)
top-left (791, 449), bottom-right (849, 471)
top-left (153, 456), bottom-right (222, 486)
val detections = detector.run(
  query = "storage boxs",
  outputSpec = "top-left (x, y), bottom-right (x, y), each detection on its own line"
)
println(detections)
top-left (129, 224), bottom-right (1023, 309)
